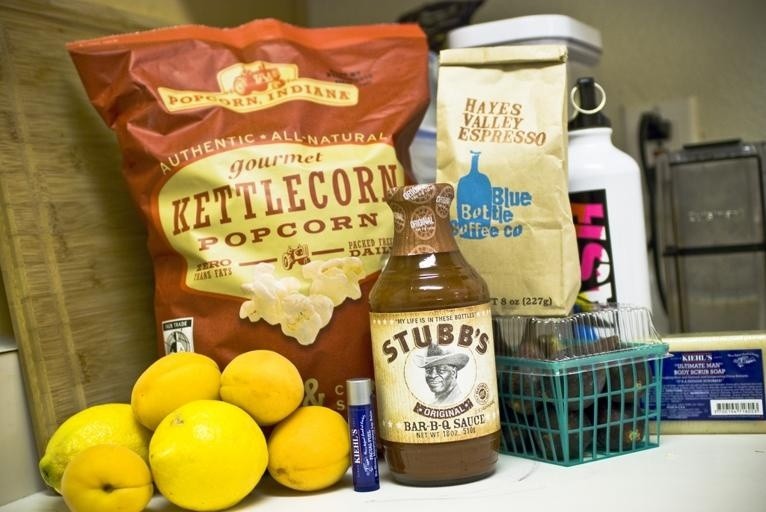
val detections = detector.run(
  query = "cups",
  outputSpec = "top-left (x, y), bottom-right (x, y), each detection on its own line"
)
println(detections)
top-left (566, 75), bottom-right (654, 343)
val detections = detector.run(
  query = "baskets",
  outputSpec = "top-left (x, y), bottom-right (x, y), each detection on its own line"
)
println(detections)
top-left (497, 338), bottom-right (667, 467)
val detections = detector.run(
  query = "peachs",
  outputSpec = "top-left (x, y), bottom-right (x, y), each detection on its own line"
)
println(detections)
top-left (130, 352), bottom-right (221, 431)
top-left (61, 443), bottom-right (153, 511)
top-left (219, 351), bottom-right (305, 426)
top-left (267, 405), bottom-right (352, 492)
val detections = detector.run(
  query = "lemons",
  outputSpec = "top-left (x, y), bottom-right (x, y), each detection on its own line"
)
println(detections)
top-left (148, 399), bottom-right (269, 511)
top-left (38, 403), bottom-right (155, 494)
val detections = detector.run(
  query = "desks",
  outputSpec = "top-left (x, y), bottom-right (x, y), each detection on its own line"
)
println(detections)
top-left (0, 429), bottom-right (766, 512)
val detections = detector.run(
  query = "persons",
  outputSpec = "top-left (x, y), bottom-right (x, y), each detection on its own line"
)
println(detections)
top-left (415, 344), bottom-right (469, 409)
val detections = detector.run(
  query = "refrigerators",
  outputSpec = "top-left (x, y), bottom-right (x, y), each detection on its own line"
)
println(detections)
top-left (655, 140), bottom-right (766, 336)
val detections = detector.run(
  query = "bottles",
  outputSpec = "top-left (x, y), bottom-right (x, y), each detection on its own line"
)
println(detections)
top-left (367, 182), bottom-right (501, 488)
